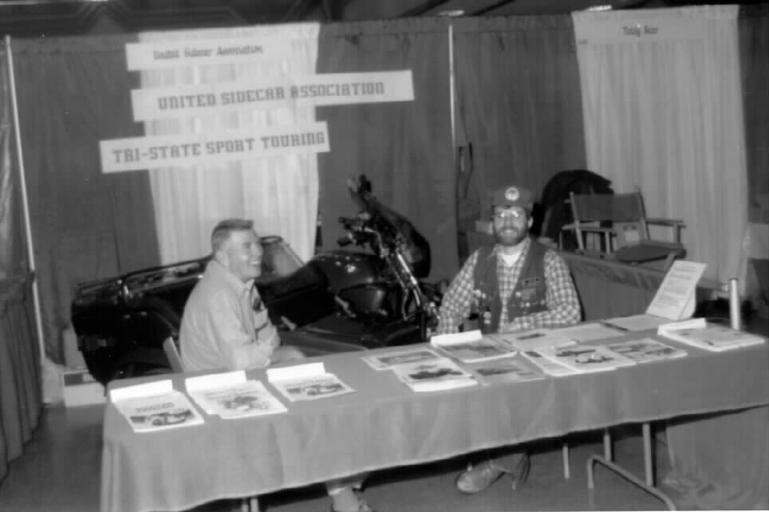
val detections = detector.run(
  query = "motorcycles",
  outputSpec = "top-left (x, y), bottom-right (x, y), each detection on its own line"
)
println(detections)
top-left (69, 169), bottom-right (479, 388)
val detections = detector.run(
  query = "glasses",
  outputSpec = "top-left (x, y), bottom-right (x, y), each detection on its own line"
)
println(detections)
top-left (494, 211), bottom-right (524, 219)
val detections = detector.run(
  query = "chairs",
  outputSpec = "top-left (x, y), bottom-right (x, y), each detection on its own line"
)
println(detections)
top-left (559, 186), bottom-right (687, 276)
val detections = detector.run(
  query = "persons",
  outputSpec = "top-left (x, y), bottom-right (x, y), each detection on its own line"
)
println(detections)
top-left (434, 184), bottom-right (585, 494)
top-left (177, 217), bottom-right (379, 512)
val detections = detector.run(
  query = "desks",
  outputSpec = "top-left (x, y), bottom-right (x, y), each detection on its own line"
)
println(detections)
top-left (107, 312), bottom-right (769, 512)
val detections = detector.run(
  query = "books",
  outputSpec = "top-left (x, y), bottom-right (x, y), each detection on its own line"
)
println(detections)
top-left (471, 360), bottom-right (544, 386)
top-left (395, 358), bottom-right (478, 392)
top-left (664, 324), bottom-right (763, 352)
top-left (438, 337), bottom-right (517, 363)
top-left (359, 347), bottom-right (441, 371)
top-left (122, 393), bottom-right (204, 429)
top-left (199, 380), bottom-right (289, 417)
top-left (495, 328), bottom-right (687, 377)
top-left (273, 374), bottom-right (356, 401)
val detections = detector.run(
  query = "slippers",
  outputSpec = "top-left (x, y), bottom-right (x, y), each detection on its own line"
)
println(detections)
top-left (331, 500), bottom-right (375, 512)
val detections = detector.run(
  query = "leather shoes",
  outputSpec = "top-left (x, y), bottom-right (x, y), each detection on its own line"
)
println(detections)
top-left (456, 460), bottom-right (505, 494)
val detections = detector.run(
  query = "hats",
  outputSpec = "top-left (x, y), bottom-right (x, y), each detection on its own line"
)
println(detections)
top-left (491, 185), bottom-right (533, 212)
